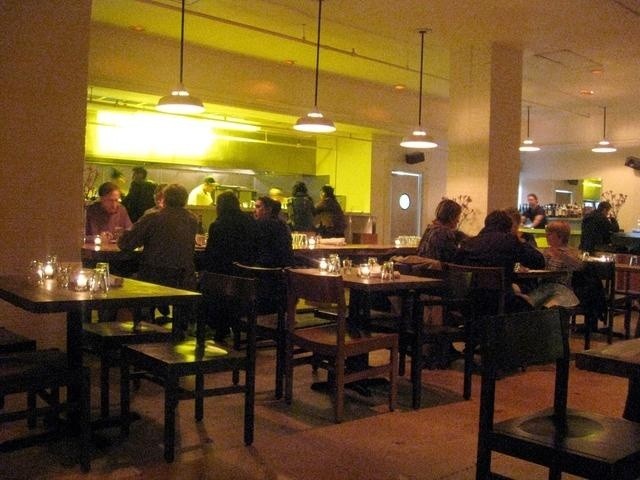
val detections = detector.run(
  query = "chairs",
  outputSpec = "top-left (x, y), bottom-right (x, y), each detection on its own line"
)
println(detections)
top-left (2, 224), bottom-right (639, 480)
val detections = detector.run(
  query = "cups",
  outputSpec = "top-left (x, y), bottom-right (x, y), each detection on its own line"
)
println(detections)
top-left (393, 235), bottom-right (421, 248)
top-left (368, 256), bottom-right (378, 272)
top-left (359, 263), bottom-right (371, 279)
top-left (382, 261), bottom-right (395, 281)
top-left (26, 256), bottom-right (110, 297)
top-left (320, 254), bottom-right (353, 276)
top-left (290, 233), bottom-right (308, 248)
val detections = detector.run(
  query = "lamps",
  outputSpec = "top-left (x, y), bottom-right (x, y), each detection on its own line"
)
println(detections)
top-left (155, 1), bottom-right (202, 116)
top-left (518, 104), bottom-right (541, 153)
top-left (403, 32), bottom-right (440, 151)
top-left (593, 103), bottom-right (616, 155)
top-left (294, 1), bottom-right (335, 133)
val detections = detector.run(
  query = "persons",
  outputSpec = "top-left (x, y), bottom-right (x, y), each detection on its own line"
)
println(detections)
top-left (580, 202), bottom-right (618, 252)
top-left (459, 211), bottom-right (548, 316)
top-left (530, 222), bottom-right (583, 313)
top-left (418, 200), bottom-right (462, 264)
top-left (521, 194), bottom-right (548, 235)
top-left (188, 176), bottom-right (345, 319)
top-left (85, 168), bottom-right (198, 319)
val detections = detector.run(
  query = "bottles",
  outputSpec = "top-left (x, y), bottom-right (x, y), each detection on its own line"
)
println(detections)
top-left (518, 203), bottom-right (581, 218)
